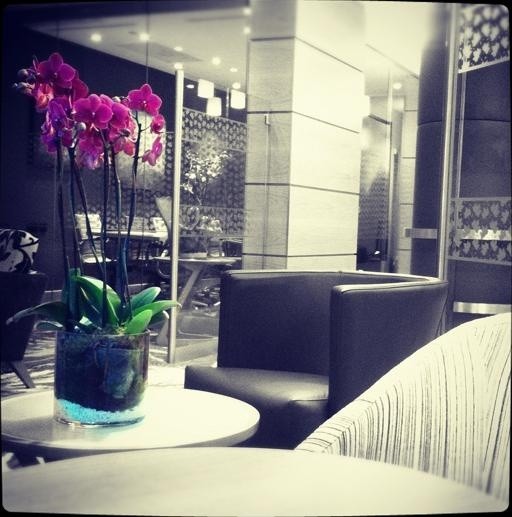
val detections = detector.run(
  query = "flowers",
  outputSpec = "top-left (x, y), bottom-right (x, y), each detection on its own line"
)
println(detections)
top-left (3, 50), bottom-right (184, 339)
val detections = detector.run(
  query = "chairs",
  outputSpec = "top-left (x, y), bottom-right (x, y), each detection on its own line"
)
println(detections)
top-left (285, 313), bottom-right (511, 487)
top-left (182, 259), bottom-right (452, 450)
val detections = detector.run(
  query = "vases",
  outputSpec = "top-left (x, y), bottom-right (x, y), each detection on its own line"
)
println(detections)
top-left (47, 328), bottom-right (152, 428)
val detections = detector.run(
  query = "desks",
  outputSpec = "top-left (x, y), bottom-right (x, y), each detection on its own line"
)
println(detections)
top-left (149, 253), bottom-right (238, 343)
top-left (3, 447), bottom-right (508, 514)
top-left (1, 388), bottom-right (268, 470)
